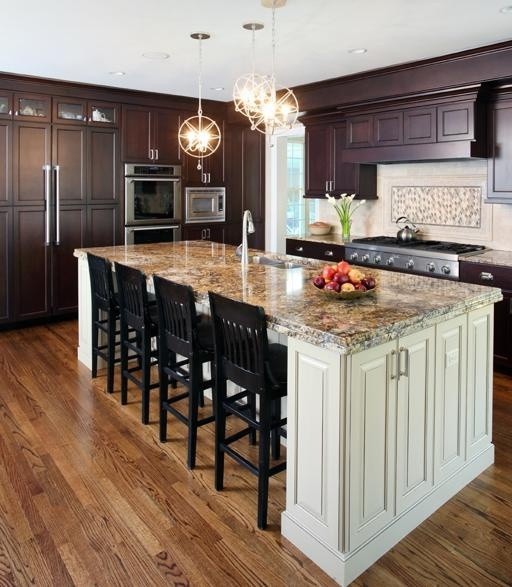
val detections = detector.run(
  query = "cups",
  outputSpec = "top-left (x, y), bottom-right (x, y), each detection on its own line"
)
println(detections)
top-left (211, 241), bottom-right (225, 258)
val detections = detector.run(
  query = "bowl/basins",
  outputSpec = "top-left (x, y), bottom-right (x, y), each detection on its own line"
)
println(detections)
top-left (310, 224), bottom-right (331, 236)
top-left (309, 280), bottom-right (379, 299)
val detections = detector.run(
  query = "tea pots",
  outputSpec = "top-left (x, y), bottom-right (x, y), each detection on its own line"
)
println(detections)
top-left (394, 215), bottom-right (420, 241)
top-left (92, 108), bottom-right (106, 122)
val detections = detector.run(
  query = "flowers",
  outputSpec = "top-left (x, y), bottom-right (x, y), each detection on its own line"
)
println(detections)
top-left (320, 189), bottom-right (366, 241)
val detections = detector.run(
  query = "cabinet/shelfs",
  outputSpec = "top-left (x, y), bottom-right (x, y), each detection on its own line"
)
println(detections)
top-left (457, 258), bottom-right (512, 381)
top-left (222, 100), bottom-right (266, 253)
top-left (285, 235), bottom-right (344, 262)
top-left (0, 71), bottom-right (123, 332)
top-left (117, 85), bottom-right (182, 166)
top-left (481, 82), bottom-right (512, 206)
top-left (295, 105), bottom-right (381, 200)
top-left (344, 324), bottom-right (435, 554)
top-left (174, 94), bottom-right (228, 185)
top-left (184, 220), bottom-right (225, 246)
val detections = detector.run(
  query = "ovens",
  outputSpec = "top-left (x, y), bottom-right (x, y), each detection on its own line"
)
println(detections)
top-left (124, 163), bottom-right (183, 243)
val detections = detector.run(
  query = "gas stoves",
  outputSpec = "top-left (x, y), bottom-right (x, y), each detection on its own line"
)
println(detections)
top-left (344, 234), bottom-right (488, 280)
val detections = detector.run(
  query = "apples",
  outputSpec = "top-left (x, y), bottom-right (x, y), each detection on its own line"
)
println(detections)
top-left (314, 262), bottom-right (376, 293)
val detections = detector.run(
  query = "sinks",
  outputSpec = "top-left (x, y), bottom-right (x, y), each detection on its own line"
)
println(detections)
top-left (273, 262), bottom-right (305, 272)
top-left (232, 253), bottom-right (279, 271)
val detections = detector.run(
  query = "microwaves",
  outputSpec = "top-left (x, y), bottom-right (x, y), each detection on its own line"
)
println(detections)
top-left (183, 183), bottom-right (229, 223)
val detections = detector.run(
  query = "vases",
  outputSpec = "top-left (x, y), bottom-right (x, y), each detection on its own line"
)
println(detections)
top-left (339, 219), bottom-right (352, 245)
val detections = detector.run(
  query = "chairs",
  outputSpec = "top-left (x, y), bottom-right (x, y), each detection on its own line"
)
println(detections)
top-left (150, 270), bottom-right (257, 470)
top-left (83, 251), bottom-right (166, 394)
top-left (113, 255), bottom-right (206, 425)
top-left (206, 285), bottom-right (293, 535)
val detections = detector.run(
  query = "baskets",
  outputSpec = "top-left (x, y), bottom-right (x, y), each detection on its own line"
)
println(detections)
top-left (311, 268), bottom-right (378, 299)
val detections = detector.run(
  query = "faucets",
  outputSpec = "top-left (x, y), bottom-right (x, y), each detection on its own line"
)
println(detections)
top-left (242, 265), bottom-right (252, 303)
top-left (241, 210), bottom-right (256, 265)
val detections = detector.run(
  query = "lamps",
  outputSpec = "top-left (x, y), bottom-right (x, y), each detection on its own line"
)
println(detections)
top-left (231, 17), bottom-right (271, 122)
top-left (246, 0), bottom-right (301, 137)
top-left (177, 30), bottom-right (224, 168)
